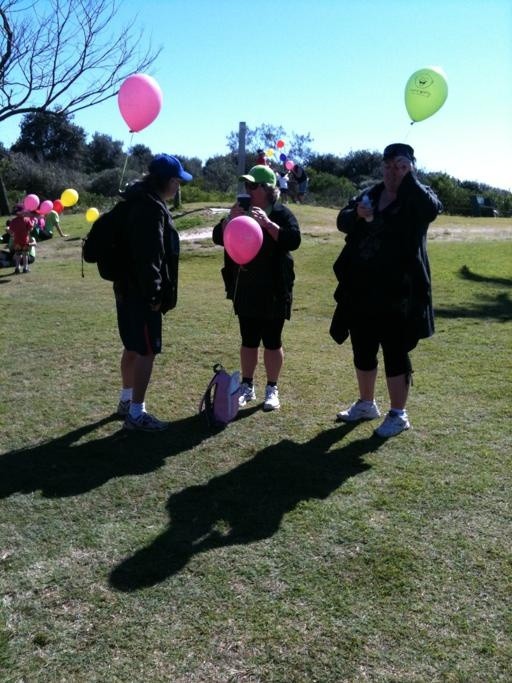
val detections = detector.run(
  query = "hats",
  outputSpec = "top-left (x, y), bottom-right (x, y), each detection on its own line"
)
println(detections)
top-left (148, 153), bottom-right (194, 183)
top-left (381, 143), bottom-right (415, 162)
top-left (239, 164), bottom-right (278, 186)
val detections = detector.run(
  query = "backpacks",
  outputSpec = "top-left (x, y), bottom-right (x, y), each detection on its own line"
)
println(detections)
top-left (80, 200), bottom-right (129, 283)
top-left (199, 363), bottom-right (241, 423)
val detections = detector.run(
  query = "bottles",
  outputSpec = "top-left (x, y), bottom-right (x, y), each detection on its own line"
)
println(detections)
top-left (361, 193), bottom-right (374, 222)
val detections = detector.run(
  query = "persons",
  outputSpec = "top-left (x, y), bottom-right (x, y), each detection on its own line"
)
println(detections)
top-left (329, 144), bottom-right (442, 440)
top-left (0, 204), bottom-right (70, 273)
top-left (257, 149), bottom-right (307, 204)
top-left (108, 152), bottom-right (194, 432)
top-left (212, 166), bottom-right (301, 412)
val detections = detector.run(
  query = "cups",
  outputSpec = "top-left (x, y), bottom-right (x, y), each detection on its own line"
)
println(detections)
top-left (236, 193), bottom-right (251, 211)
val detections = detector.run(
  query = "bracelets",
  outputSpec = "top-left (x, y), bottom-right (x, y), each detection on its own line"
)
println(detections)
top-left (266, 221), bottom-right (274, 230)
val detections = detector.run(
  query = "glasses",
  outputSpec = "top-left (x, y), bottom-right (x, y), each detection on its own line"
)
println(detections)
top-left (243, 181), bottom-right (263, 191)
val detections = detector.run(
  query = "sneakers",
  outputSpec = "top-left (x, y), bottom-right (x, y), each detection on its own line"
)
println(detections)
top-left (122, 411), bottom-right (170, 432)
top-left (336, 397), bottom-right (381, 422)
top-left (237, 382), bottom-right (257, 408)
top-left (262, 384), bottom-right (281, 412)
top-left (374, 411), bottom-right (411, 439)
top-left (117, 400), bottom-right (132, 416)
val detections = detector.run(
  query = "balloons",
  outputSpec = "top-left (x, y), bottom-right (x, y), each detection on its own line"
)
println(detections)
top-left (118, 73), bottom-right (162, 132)
top-left (267, 140), bottom-right (295, 170)
top-left (23, 187), bottom-right (80, 216)
top-left (222, 215), bottom-right (264, 266)
top-left (85, 208), bottom-right (100, 223)
top-left (405, 68), bottom-right (448, 125)
